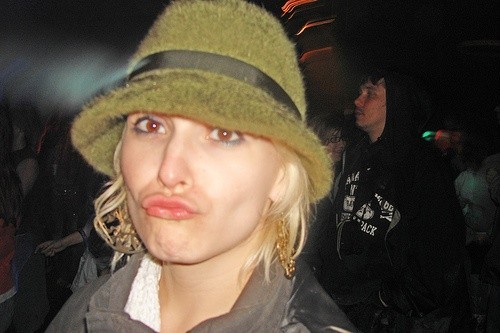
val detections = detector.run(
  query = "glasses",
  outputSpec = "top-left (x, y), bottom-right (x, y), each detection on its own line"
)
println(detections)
top-left (325, 137), bottom-right (344, 146)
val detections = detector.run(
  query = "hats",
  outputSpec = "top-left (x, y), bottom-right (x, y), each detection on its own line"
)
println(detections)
top-left (70, 0), bottom-right (336, 204)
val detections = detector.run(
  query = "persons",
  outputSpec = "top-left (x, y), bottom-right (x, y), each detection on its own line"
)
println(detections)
top-left (315, 62), bottom-right (465, 333)
top-left (301, 112), bottom-right (349, 264)
top-left (45, 0), bottom-right (358, 333)
top-left (0, 101), bottom-right (40, 333)
top-left (16, 98), bottom-right (112, 333)
top-left (443, 111), bottom-right (500, 333)
